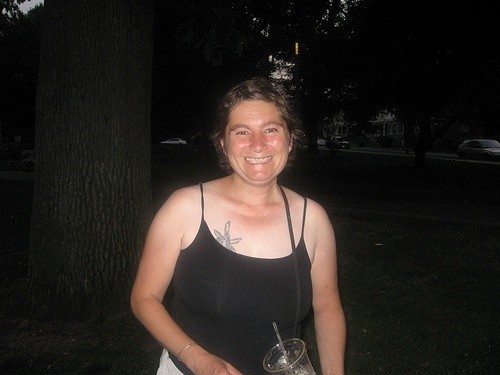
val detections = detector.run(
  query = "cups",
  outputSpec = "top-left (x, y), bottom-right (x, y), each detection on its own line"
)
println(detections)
top-left (330, 115), bottom-right (345, 139)
top-left (263, 338), bottom-right (315, 375)
top-left (386, 122), bottom-right (405, 139)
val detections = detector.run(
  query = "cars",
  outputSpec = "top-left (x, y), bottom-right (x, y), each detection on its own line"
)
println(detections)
top-left (159, 137), bottom-right (187, 146)
top-left (329, 135), bottom-right (350, 149)
top-left (316, 136), bottom-right (326, 146)
top-left (457, 139), bottom-right (500, 161)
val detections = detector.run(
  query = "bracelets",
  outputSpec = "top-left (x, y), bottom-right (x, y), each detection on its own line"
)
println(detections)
top-left (177, 342), bottom-right (198, 362)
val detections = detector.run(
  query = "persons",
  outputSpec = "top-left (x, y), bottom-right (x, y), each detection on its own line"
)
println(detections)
top-left (129, 76), bottom-right (347, 375)
